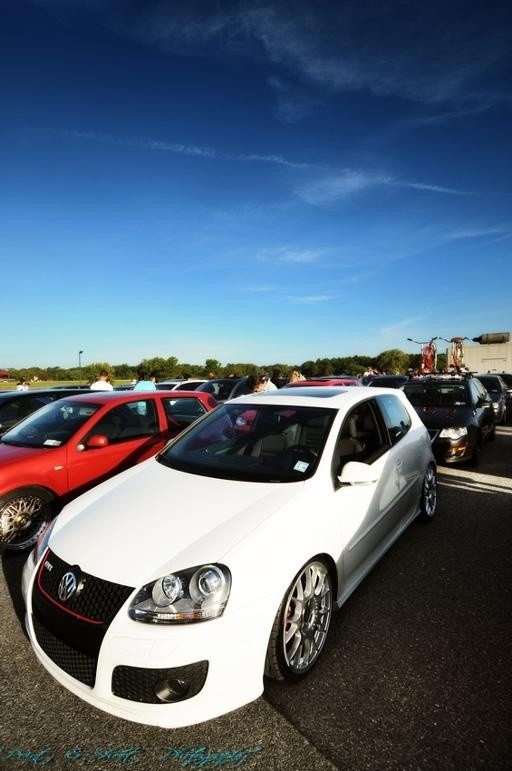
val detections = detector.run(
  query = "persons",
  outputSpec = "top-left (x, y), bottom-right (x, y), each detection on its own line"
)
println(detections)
top-left (16, 379), bottom-right (26, 391)
top-left (149, 373), bottom-right (158, 384)
top-left (89, 371), bottom-right (113, 392)
top-left (25, 383), bottom-right (30, 390)
top-left (227, 372), bottom-right (236, 378)
top-left (126, 366), bottom-right (156, 416)
top-left (247, 367), bottom-right (306, 394)
top-left (207, 371), bottom-right (216, 380)
top-left (357, 362), bottom-right (458, 380)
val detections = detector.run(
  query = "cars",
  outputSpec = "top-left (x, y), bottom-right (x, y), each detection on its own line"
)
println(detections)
top-left (20, 386), bottom-right (438, 729)
top-left (1, 389), bottom-right (218, 554)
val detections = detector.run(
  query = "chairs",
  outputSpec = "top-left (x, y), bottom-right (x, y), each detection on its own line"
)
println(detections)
top-left (93, 414), bottom-right (155, 440)
top-left (252, 414), bottom-right (365, 475)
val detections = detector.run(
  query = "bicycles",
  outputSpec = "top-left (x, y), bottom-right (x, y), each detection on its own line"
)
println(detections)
top-left (441, 337), bottom-right (469, 374)
top-left (409, 337), bottom-right (438, 375)
